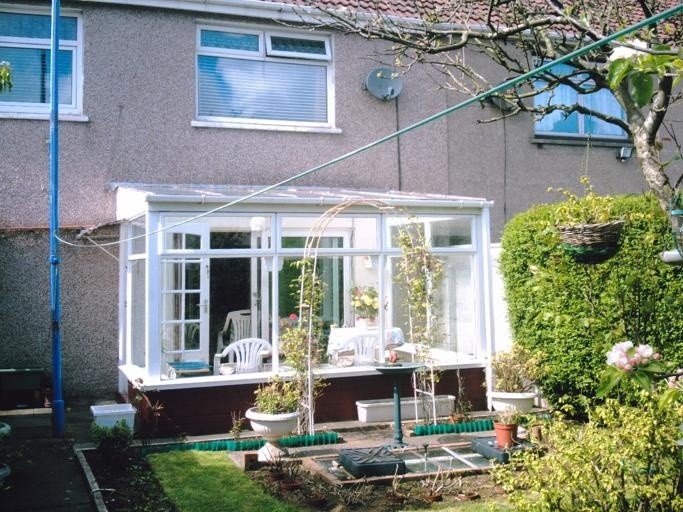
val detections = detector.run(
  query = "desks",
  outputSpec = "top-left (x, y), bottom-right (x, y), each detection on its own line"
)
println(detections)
top-left (325, 326), bottom-right (403, 355)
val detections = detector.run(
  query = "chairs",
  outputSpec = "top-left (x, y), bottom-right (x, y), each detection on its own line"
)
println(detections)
top-left (327, 334), bottom-right (380, 365)
top-left (213, 308), bottom-right (272, 374)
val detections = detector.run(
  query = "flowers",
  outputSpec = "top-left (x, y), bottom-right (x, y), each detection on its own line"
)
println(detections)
top-left (349, 283), bottom-right (389, 323)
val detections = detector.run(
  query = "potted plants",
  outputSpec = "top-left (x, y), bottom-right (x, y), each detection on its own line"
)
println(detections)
top-left (245, 377), bottom-right (306, 434)
top-left (539, 175), bottom-right (654, 263)
top-left (484, 344), bottom-right (542, 447)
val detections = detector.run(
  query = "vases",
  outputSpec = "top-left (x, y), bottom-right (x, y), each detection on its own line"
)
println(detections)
top-left (90, 404), bottom-right (137, 436)
top-left (353, 317), bottom-right (379, 329)
